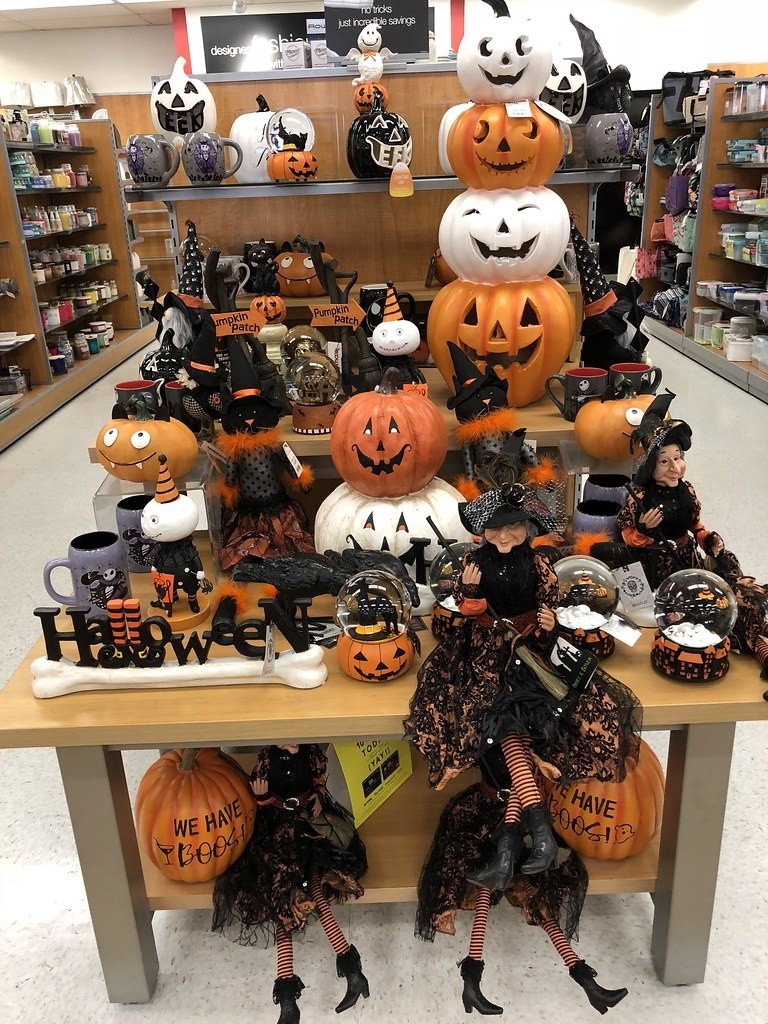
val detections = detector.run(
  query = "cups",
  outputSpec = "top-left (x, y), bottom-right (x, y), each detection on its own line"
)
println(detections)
top-left (359, 284), bottom-right (416, 332)
top-left (544, 367), bottom-right (608, 422)
top-left (100, 243), bottom-right (112, 261)
top-left (586, 113), bottom-right (634, 164)
top-left (181, 131), bottom-right (243, 185)
top-left (202, 255), bottom-right (251, 302)
top-left (77, 207), bottom-right (98, 228)
top-left (37, 296), bottom-right (93, 331)
top-left (48, 320), bottom-right (115, 375)
top-left (124, 133), bottom-right (180, 188)
top-left (572, 472), bottom-right (633, 535)
top-left (28, 244), bottom-right (99, 285)
top-left (113, 379), bottom-right (201, 433)
top-left (559, 242), bottom-right (600, 285)
top-left (115, 493), bottom-right (156, 574)
top-left (42, 529), bottom-right (131, 620)
top-left (610, 362), bottom-right (662, 400)
top-left (241, 240), bottom-right (279, 293)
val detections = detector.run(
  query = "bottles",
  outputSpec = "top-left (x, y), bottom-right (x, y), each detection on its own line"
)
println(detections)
top-left (38, 163), bottom-right (92, 189)
top-left (11, 151), bottom-right (54, 188)
top-left (58, 279), bottom-right (118, 298)
top-left (32, 120), bottom-right (81, 146)
top-left (22, 204), bottom-right (79, 233)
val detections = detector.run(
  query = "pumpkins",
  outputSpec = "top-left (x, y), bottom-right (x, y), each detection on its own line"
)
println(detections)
top-left (96, 396), bottom-right (197, 483)
top-left (228, 94), bottom-right (285, 184)
top-left (329, 365), bottom-right (447, 499)
top-left (573, 381), bottom-right (674, 461)
top-left (270, 235), bottom-right (337, 298)
top-left (540, 732), bottom-right (666, 861)
top-left (133, 747), bottom-right (256, 884)
top-left (336, 622), bottom-right (414, 683)
top-left (314, 476), bottom-right (476, 617)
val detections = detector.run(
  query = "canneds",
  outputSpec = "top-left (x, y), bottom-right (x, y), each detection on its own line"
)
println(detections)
top-left (48, 321), bottom-right (114, 376)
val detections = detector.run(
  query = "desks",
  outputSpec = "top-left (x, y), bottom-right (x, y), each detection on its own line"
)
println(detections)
top-left (279, 340), bottom-right (598, 496)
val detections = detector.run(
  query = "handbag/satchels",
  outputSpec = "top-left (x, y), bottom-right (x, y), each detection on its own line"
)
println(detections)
top-left (617, 68), bottom-right (736, 330)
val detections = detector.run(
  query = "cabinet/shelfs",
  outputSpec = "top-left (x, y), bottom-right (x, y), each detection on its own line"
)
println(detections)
top-left (123, 162), bottom-right (642, 308)
top-left (641, 79), bottom-right (768, 406)
top-left (0, 114), bottom-right (157, 453)
top-left (0, 539), bottom-right (768, 1005)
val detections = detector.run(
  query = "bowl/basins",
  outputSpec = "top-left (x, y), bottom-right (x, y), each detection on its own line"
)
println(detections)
top-left (725, 127), bottom-right (768, 163)
top-left (709, 183), bottom-right (768, 214)
